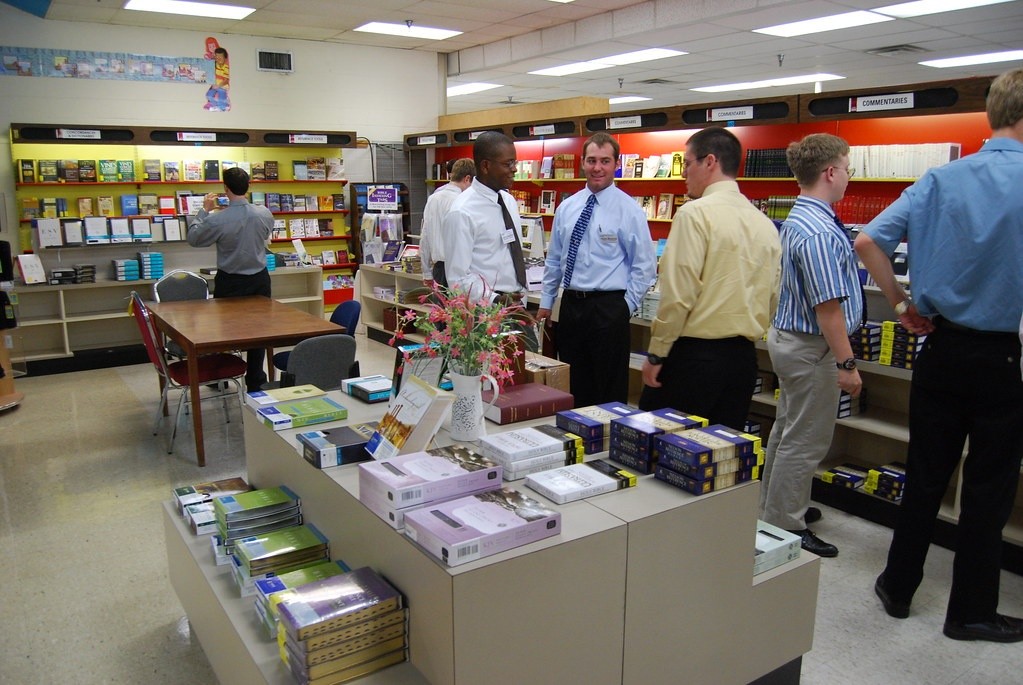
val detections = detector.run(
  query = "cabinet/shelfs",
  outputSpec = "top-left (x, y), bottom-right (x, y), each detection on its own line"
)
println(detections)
top-left (423, 171), bottom-right (937, 226)
top-left (360, 267), bottom-right (439, 344)
top-left (1, 267), bottom-right (325, 363)
top-left (161, 386), bottom-right (628, 684)
top-left (538, 278), bottom-right (1022, 588)
top-left (442, 386), bottom-right (821, 685)
top-left (21, 174), bottom-right (357, 277)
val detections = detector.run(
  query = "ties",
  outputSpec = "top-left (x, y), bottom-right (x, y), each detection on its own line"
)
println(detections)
top-left (563, 194), bottom-right (597, 288)
top-left (834, 214), bottom-right (867, 326)
top-left (497, 192), bottom-right (527, 289)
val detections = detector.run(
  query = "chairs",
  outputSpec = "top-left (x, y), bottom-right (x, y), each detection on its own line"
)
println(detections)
top-left (273, 300), bottom-right (364, 373)
top-left (133, 291), bottom-right (248, 453)
top-left (285, 333), bottom-right (357, 389)
top-left (155, 268), bottom-right (247, 416)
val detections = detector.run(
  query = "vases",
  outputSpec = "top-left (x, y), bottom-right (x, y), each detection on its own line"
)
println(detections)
top-left (438, 366), bottom-right (498, 440)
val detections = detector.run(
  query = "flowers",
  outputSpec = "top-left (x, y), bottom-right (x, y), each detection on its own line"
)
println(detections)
top-left (396, 281), bottom-right (536, 385)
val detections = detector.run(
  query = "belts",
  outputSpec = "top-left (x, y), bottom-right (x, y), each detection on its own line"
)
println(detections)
top-left (564, 289), bottom-right (627, 299)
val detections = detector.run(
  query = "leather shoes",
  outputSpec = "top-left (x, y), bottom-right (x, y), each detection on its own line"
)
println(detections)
top-left (943, 611), bottom-right (1023, 643)
top-left (875, 571), bottom-right (909, 619)
top-left (786, 528), bottom-right (839, 558)
top-left (803, 507), bottom-right (821, 524)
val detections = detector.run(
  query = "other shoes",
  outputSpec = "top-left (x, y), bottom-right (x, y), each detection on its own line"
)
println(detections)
top-left (206, 380), bottom-right (228, 388)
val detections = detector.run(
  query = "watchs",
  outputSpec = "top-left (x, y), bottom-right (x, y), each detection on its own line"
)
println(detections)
top-left (895, 298), bottom-right (911, 315)
top-left (647, 354), bottom-right (668, 365)
top-left (833, 359), bottom-right (857, 371)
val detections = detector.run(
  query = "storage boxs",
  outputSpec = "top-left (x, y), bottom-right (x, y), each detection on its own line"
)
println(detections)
top-left (524, 351), bottom-right (571, 394)
top-left (323, 285), bottom-right (355, 303)
top-left (384, 308), bottom-right (414, 332)
top-left (171, 250), bottom-right (923, 685)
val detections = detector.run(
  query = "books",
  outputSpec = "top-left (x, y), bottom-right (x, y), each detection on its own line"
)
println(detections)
top-left (528, 459), bottom-right (639, 504)
top-left (365, 371), bottom-right (456, 463)
top-left (0, 140), bottom-right (1023, 685)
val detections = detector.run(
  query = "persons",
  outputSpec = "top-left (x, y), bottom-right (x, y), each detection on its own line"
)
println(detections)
top-left (185, 167), bottom-right (275, 393)
top-left (641, 125), bottom-right (780, 432)
top-left (419, 130), bottom-right (529, 313)
top-left (768, 134), bottom-right (863, 559)
top-left (855, 70), bottom-right (1023, 642)
top-left (535, 132), bottom-right (657, 410)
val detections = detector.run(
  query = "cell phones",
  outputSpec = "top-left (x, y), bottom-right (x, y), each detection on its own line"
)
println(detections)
top-left (217, 195), bottom-right (230, 206)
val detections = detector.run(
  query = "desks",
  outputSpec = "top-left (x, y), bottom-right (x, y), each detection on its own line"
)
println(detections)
top-left (143, 295), bottom-right (345, 467)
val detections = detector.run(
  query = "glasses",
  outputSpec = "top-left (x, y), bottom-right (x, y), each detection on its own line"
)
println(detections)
top-left (682, 155), bottom-right (717, 168)
top-left (479, 157), bottom-right (518, 168)
top-left (822, 166), bottom-right (856, 180)
